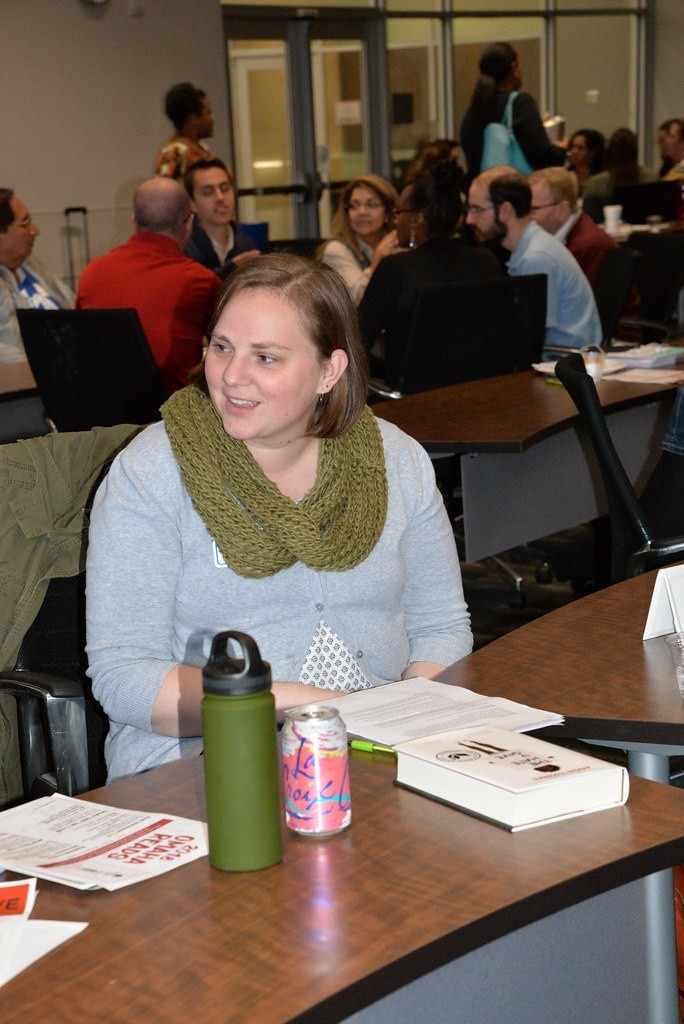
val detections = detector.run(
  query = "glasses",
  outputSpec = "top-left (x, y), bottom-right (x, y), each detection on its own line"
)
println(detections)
top-left (348, 198), bottom-right (384, 210)
top-left (530, 201), bottom-right (572, 215)
top-left (394, 209), bottom-right (428, 224)
top-left (466, 205), bottom-right (498, 215)
top-left (10, 217), bottom-right (32, 229)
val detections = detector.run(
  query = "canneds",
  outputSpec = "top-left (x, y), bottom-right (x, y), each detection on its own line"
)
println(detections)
top-left (280, 705), bottom-right (352, 837)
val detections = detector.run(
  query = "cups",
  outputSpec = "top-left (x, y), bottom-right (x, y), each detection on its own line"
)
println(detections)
top-left (646, 215), bottom-right (661, 233)
top-left (663, 632), bottom-right (684, 695)
top-left (604, 205), bottom-right (622, 235)
top-left (579, 343), bottom-right (605, 383)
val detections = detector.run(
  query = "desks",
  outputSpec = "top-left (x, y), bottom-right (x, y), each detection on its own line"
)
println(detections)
top-left (0, 724), bottom-right (684, 1024)
top-left (419, 563), bottom-right (684, 791)
top-left (369, 348), bottom-right (684, 584)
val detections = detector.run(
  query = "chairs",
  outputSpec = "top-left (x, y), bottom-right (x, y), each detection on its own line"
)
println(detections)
top-left (0, 178), bottom-right (684, 809)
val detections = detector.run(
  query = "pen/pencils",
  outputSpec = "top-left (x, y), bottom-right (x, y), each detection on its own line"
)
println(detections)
top-left (544, 378), bottom-right (563, 386)
top-left (348, 739), bottom-right (395, 754)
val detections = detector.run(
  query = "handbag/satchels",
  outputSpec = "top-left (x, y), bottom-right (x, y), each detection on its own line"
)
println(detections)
top-left (481, 91), bottom-right (533, 176)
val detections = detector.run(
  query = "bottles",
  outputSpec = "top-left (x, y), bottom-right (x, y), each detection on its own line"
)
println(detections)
top-left (201, 631), bottom-right (284, 873)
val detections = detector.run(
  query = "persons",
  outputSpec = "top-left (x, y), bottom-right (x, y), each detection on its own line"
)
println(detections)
top-left (153, 81), bottom-right (236, 211)
top-left (581, 128), bottom-right (660, 198)
top-left (0, 188), bottom-right (78, 447)
top-left (357, 157), bottom-right (507, 396)
top-left (311, 172), bottom-right (416, 311)
top-left (527, 165), bottom-right (628, 340)
top-left (654, 118), bottom-right (684, 215)
top-left (184, 157), bottom-right (270, 287)
top-left (401, 138), bottom-right (468, 189)
top-left (466, 164), bottom-right (604, 363)
top-left (461, 45), bottom-right (552, 177)
top-left (565, 131), bottom-right (608, 199)
top-left (74, 175), bottom-right (228, 409)
top-left (86, 255), bottom-right (477, 782)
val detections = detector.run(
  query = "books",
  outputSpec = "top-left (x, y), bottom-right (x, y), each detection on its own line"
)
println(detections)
top-left (391, 725), bottom-right (631, 831)
